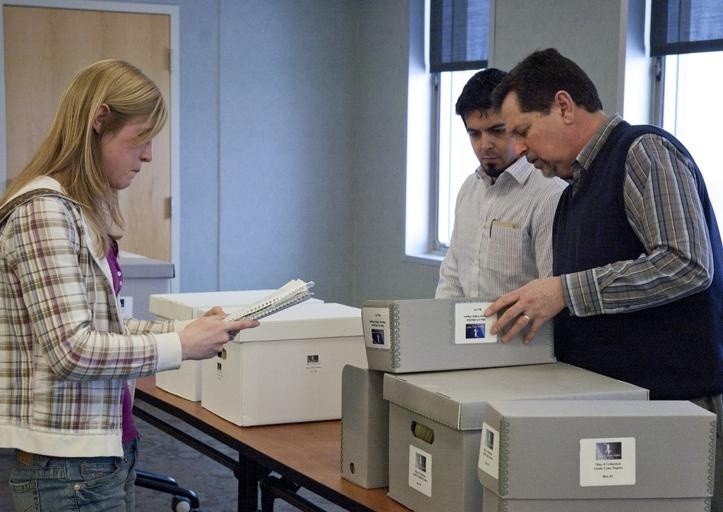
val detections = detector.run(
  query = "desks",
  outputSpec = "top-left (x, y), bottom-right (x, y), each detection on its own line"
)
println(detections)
top-left (134, 375), bottom-right (404, 508)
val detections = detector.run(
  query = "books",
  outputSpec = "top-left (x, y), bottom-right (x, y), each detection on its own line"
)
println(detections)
top-left (223, 278), bottom-right (314, 327)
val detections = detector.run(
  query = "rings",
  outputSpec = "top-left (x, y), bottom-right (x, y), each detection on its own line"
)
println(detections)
top-left (522, 313), bottom-right (530, 323)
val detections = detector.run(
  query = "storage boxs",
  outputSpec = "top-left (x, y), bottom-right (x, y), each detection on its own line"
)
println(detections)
top-left (116, 251), bottom-right (176, 322)
top-left (149, 290), bottom-right (368, 428)
top-left (339, 298), bottom-right (717, 512)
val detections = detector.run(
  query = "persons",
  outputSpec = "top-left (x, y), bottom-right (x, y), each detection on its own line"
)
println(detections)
top-left (429, 68), bottom-right (571, 302)
top-left (0, 58), bottom-right (261, 511)
top-left (482, 48), bottom-right (721, 511)
